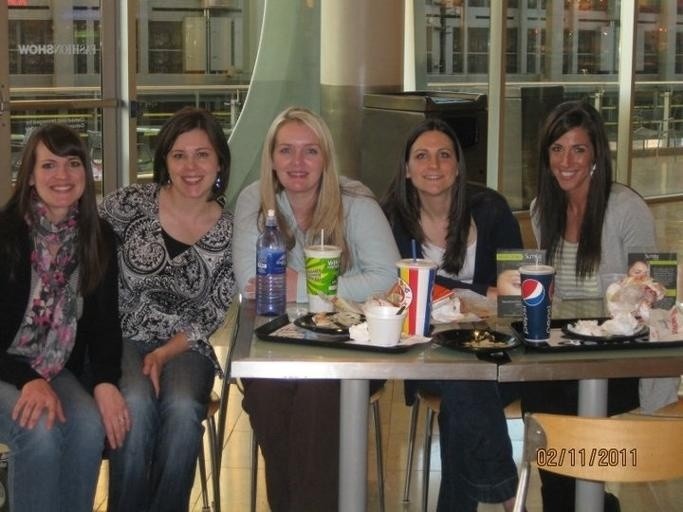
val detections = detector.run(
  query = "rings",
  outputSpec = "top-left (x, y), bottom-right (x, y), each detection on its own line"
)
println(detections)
top-left (116, 417), bottom-right (125, 424)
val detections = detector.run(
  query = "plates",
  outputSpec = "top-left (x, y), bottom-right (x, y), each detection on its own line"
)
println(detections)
top-left (292, 311), bottom-right (367, 335)
top-left (429, 328), bottom-right (522, 354)
top-left (560, 320), bottom-right (651, 342)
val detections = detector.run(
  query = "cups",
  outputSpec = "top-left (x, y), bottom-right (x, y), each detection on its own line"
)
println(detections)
top-left (396, 258), bottom-right (438, 335)
top-left (365, 306), bottom-right (407, 346)
top-left (302, 245), bottom-right (343, 312)
top-left (518, 264), bottom-right (556, 343)
top-left (598, 272), bottom-right (629, 317)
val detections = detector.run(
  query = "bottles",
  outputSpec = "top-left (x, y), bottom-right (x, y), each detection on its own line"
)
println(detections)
top-left (256, 208), bottom-right (286, 318)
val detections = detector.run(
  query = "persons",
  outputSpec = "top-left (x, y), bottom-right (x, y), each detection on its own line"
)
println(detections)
top-left (528, 100), bottom-right (657, 512)
top-left (0, 125), bottom-right (132, 512)
top-left (230, 106), bottom-right (401, 512)
top-left (626, 258), bottom-right (676, 310)
top-left (96, 106), bottom-right (235, 512)
top-left (377, 117), bottom-right (524, 512)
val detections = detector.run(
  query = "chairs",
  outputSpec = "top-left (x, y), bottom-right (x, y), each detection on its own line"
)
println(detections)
top-left (512, 207), bottom-right (539, 252)
top-left (0, 443), bottom-right (10, 512)
top-left (95, 390), bottom-right (226, 511)
top-left (510, 410), bottom-right (682, 512)
